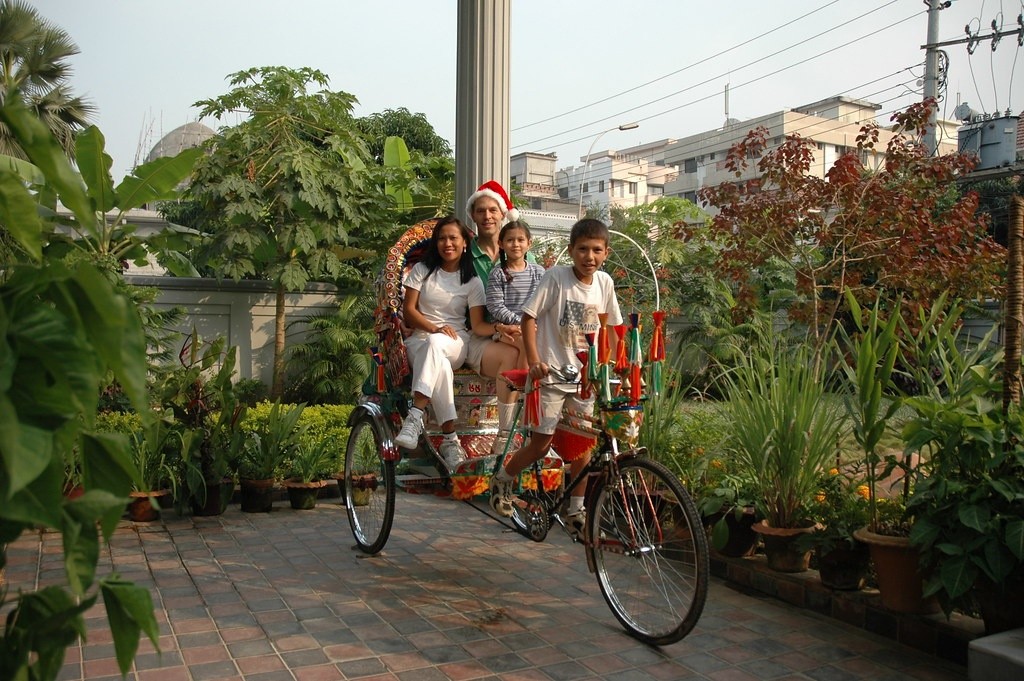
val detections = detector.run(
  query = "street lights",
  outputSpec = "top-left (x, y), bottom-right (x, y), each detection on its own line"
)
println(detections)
top-left (576, 122), bottom-right (640, 224)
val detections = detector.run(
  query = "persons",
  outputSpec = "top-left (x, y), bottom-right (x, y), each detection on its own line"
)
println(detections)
top-left (487, 219), bottom-right (624, 542)
top-left (485, 220), bottom-right (545, 370)
top-left (393, 216), bottom-right (521, 471)
top-left (465, 181), bottom-right (538, 457)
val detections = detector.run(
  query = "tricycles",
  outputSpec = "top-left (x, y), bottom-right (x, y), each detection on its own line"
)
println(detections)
top-left (343, 217), bottom-right (712, 647)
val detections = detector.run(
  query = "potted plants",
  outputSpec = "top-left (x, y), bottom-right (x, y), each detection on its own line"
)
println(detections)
top-left (62, 449), bottom-right (84, 502)
top-left (615, 328), bottom-right (708, 530)
top-left (693, 459), bottom-right (762, 577)
top-left (235, 396), bottom-right (313, 513)
top-left (333, 435), bottom-right (414, 506)
top-left (704, 297), bottom-right (856, 573)
top-left (899, 363), bottom-right (1024, 635)
top-left (283, 429), bottom-right (333, 509)
top-left (111, 407), bottom-right (192, 522)
top-left (810, 452), bottom-right (895, 590)
top-left (160, 322), bottom-right (247, 516)
top-left (836, 282), bottom-right (1007, 614)
top-left (658, 451), bottom-right (717, 540)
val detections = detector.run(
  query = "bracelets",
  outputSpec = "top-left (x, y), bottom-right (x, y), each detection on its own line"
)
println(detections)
top-left (494, 321), bottom-right (504, 333)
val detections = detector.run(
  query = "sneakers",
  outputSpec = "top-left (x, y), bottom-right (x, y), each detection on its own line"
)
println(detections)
top-left (394, 406), bottom-right (424, 449)
top-left (489, 471), bottom-right (515, 518)
top-left (439, 431), bottom-right (468, 469)
top-left (564, 506), bottom-right (606, 543)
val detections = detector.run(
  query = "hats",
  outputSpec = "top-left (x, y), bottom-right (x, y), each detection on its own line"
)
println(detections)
top-left (465, 181), bottom-right (520, 222)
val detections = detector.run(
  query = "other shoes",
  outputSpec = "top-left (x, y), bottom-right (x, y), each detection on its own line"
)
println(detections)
top-left (491, 430), bottom-right (515, 454)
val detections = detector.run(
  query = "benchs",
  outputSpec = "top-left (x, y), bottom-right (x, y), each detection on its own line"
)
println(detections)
top-left (374, 216), bottom-right (525, 434)
